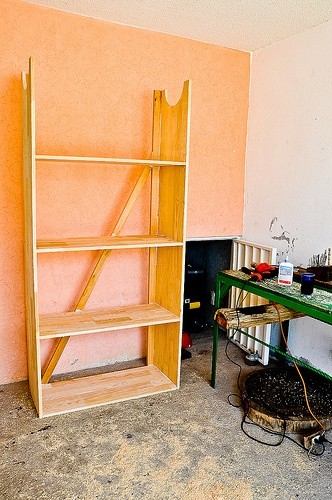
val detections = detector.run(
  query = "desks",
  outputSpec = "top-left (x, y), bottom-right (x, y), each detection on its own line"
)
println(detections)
top-left (211, 265), bottom-right (332, 388)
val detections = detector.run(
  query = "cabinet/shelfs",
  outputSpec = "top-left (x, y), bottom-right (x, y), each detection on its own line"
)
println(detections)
top-left (19, 56), bottom-right (192, 420)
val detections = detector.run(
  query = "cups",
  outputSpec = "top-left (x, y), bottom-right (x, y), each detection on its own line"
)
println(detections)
top-left (300, 273), bottom-right (315, 295)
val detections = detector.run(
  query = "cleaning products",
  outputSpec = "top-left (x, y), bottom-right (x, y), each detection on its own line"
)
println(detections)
top-left (277, 256), bottom-right (293, 286)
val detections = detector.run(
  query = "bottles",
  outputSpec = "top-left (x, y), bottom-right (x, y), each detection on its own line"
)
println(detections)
top-left (278, 256), bottom-right (294, 286)
